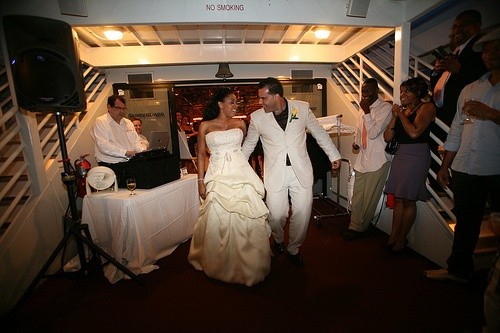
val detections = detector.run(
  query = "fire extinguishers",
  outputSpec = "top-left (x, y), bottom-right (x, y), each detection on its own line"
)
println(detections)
top-left (75, 154), bottom-right (91, 198)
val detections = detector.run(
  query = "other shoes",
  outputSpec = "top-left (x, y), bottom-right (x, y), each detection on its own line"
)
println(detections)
top-left (422, 267), bottom-right (468, 283)
top-left (273, 240), bottom-right (285, 255)
top-left (338, 227), bottom-right (370, 241)
top-left (287, 251), bottom-right (304, 268)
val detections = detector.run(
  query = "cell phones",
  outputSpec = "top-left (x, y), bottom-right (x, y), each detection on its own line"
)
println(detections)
top-left (353, 145), bottom-right (359, 150)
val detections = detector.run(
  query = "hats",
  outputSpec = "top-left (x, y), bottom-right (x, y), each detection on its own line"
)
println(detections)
top-left (474, 28), bottom-right (500, 45)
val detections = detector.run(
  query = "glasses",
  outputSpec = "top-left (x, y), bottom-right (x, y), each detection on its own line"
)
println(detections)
top-left (110, 104), bottom-right (127, 111)
top-left (449, 21), bottom-right (474, 30)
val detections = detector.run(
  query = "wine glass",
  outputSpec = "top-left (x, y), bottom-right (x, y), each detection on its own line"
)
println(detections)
top-left (126, 178), bottom-right (138, 196)
top-left (460, 96), bottom-right (481, 123)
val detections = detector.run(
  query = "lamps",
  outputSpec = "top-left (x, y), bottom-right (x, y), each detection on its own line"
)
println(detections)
top-left (103, 27), bottom-right (127, 41)
top-left (311, 27), bottom-right (332, 39)
top-left (214, 64), bottom-right (233, 81)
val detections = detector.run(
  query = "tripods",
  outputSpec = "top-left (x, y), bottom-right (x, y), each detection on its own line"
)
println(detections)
top-left (9, 111), bottom-right (146, 317)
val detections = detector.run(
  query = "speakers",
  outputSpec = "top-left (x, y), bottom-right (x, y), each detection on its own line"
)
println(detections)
top-left (5, 14), bottom-right (87, 113)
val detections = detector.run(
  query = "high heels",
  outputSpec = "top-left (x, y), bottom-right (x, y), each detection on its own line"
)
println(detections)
top-left (383, 238), bottom-right (409, 257)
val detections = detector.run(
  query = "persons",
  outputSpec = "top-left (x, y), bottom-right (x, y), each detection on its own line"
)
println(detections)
top-left (422, 23), bottom-right (500, 283)
top-left (240, 77), bottom-right (342, 268)
top-left (91, 95), bottom-right (198, 185)
top-left (385, 76), bottom-right (436, 257)
top-left (188, 88), bottom-right (273, 292)
top-left (339, 78), bottom-right (393, 240)
top-left (429, 10), bottom-right (488, 219)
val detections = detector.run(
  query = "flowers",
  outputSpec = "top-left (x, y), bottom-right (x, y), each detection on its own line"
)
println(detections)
top-left (289, 106), bottom-right (299, 122)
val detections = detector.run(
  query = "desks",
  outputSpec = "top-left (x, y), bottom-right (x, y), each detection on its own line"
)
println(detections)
top-left (63, 174), bottom-right (199, 283)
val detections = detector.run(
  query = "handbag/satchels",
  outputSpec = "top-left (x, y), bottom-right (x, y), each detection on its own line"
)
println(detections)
top-left (385, 138), bottom-right (398, 155)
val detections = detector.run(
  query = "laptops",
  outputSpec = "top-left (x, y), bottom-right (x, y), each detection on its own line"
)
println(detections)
top-left (134, 130), bottom-right (171, 154)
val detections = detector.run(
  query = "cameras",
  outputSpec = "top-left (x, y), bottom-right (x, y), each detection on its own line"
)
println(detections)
top-left (385, 139), bottom-right (398, 154)
top-left (432, 45), bottom-right (463, 74)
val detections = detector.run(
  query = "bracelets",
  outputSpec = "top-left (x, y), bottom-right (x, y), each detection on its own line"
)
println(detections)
top-left (197, 178), bottom-right (205, 181)
